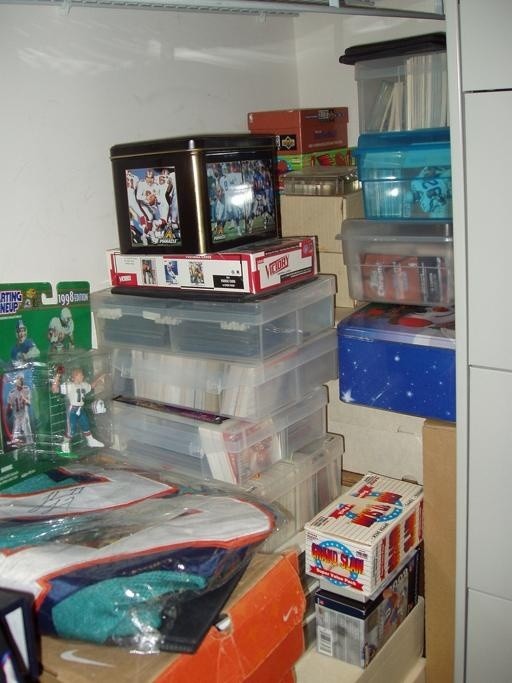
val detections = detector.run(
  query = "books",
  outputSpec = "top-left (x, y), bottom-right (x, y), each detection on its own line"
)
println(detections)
top-left (348, 147), bottom-right (455, 306)
top-left (101, 291), bottom-right (343, 557)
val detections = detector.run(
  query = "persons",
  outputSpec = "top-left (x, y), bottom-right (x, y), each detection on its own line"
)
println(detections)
top-left (125, 166), bottom-right (182, 247)
top-left (20, 415), bottom-right (23, 416)
top-left (6, 373), bottom-right (34, 446)
top-left (49, 363), bottom-right (107, 454)
top-left (10, 318), bottom-right (41, 367)
top-left (143, 259), bottom-right (205, 286)
top-left (205, 158), bottom-right (277, 241)
top-left (47, 307), bottom-right (79, 350)
